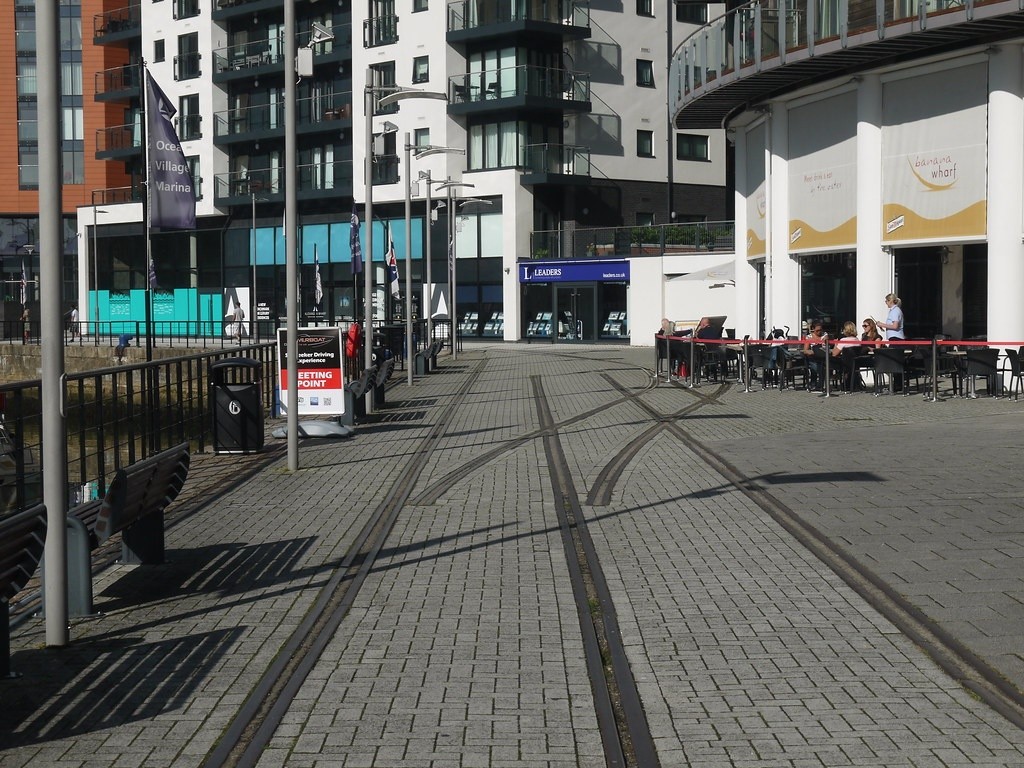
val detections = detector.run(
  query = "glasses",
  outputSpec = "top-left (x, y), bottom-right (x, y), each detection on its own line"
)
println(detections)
top-left (862, 325), bottom-right (869, 328)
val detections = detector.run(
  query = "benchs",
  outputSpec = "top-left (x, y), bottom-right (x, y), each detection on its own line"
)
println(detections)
top-left (366, 358), bottom-right (396, 412)
top-left (340, 364), bottom-right (380, 427)
top-left (0, 502), bottom-right (49, 677)
top-left (430, 338), bottom-right (445, 371)
top-left (414, 341), bottom-right (439, 375)
top-left (67, 440), bottom-right (190, 612)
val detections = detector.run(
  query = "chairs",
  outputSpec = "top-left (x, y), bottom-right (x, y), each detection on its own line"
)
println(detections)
top-left (217, 54), bottom-right (266, 71)
top-left (486, 82), bottom-right (497, 99)
top-left (657, 327), bottom-right (1024, 401)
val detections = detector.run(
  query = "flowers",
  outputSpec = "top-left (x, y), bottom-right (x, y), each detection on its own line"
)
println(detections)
top-left (586, 242), bottom-right (595, 252)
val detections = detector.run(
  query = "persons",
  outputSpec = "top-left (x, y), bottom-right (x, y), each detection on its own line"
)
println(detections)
top-left (876, 293), bottom-right (906, 392)
top-left (20, 302), bottom-right (32, 343)
top-left (69, 304), bottom-right (82, 342)
top-left (804, 317), bottom-right (883, 392)
top-left (233, 302), bottom-right (245, 344)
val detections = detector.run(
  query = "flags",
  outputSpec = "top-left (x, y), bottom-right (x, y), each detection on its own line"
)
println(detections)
top-left (384, 221), bottom-right (400, 300)
top-left (144, 63), bottom-right (197, 231)
top-left (148, 240), bottom-right (158, 289)
top-left (349, 202), bottom-right (363, 273)
top-left (315, 244), bottom-right (323, 305)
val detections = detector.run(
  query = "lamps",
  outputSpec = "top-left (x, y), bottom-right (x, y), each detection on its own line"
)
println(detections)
top-left (800, 259), bottom-right (809, 274)
top-left (936, 246), bottom-right (954, 265)
top-left (843, 253), bottom-right (856, 269)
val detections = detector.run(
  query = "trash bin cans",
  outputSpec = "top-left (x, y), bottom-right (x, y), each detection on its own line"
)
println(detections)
top-left (210, 357), bottom-right (264, 454)
top-left (354, 333), bottom-right (386, 412)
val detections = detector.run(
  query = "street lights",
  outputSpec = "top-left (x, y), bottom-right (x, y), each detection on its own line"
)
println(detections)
top-left (404, 130), bottom-right (467, 389)
top-left (362, 66), bottom-right (450, 418)
top-left (418, 168), bottom-right (474, 371)
top-left (435, 181), bottom-right (475, 364)
top-left (452, 188), bottom-right (494, 362)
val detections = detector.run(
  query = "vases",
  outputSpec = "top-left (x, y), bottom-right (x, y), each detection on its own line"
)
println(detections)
top-left (603, 252), bottom-right (613, 256)
top-left (587, 252), bottom-right (596, 257)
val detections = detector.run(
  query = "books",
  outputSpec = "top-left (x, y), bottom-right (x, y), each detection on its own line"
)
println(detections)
top-left (870, 314), bottom-right (885, 332)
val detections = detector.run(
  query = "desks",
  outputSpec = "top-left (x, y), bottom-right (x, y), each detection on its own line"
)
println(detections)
top-left (719, 345), bottom-right (744, 385)
top-left (801, 350), bottom-right (875, 393)
top-left (947, 350), bottom-right (967, 398)
top-left (459, 86), bottom-right (480, 101)
